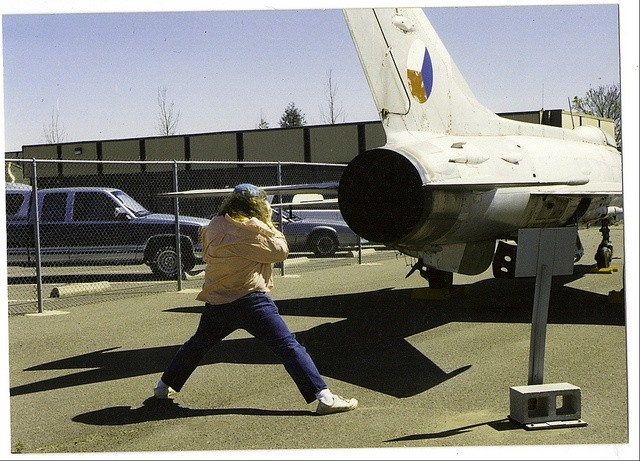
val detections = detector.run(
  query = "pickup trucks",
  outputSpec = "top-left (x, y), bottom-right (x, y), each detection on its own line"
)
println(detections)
top-left (6, 186), bottom-right (211, 281)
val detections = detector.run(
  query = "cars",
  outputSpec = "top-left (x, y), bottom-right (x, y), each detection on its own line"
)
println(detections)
top-left (267, 205), bottom-right (358, 257)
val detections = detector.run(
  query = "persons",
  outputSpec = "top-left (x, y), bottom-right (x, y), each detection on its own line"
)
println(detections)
top-left (152, 181), bottom-right (360, 417)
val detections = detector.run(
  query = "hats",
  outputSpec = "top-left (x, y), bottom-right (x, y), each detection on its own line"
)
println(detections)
top-left (233, 183), bottom-right (261, 196)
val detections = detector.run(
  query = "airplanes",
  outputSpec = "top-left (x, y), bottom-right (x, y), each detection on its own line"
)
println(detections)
top-left (158, 9), bottom-right (623, 291)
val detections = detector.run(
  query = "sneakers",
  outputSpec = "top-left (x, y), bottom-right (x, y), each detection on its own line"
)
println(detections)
top-left (316, 395), bottom-right (359, 415)
top-left (154, 380), bottom-right (176, 401)
